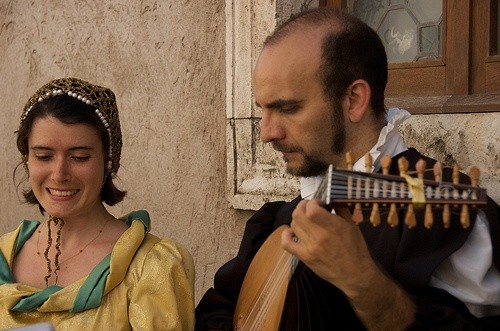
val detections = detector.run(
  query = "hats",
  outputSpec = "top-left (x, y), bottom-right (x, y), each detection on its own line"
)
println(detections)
top-left (18, 78), bottom-right (123, 181)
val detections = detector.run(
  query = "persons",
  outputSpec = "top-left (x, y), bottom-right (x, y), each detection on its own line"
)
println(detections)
top-left (0, 78), bottom-right (195, 331)
top-left (194, 8), bottom-right (500, 331)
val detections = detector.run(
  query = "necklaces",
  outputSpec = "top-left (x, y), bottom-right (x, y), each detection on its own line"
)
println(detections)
top-left (36, 219), bottom-right (111, 268)
